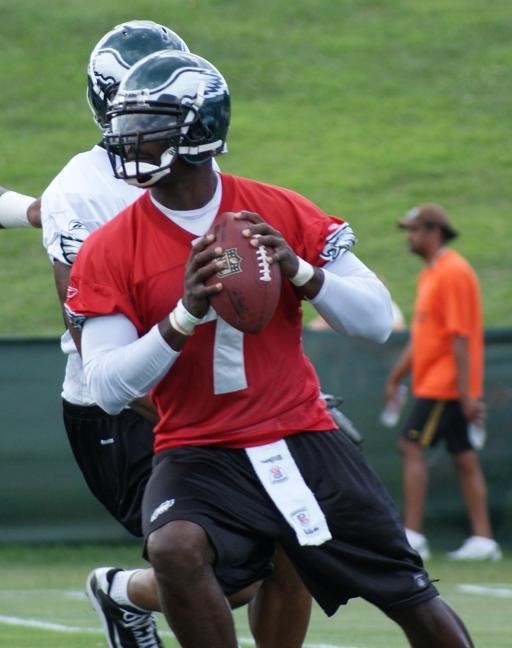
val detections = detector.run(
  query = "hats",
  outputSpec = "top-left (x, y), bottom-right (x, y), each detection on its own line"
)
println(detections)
top-left (400, 204), bottom-right (448, 231)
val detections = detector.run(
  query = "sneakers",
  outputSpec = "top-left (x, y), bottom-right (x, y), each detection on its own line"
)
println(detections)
top-left (449, 536), bottom-right (503, 563)
top-left (406, 528), bottom-right (432, 562)
top-left (85, 566), bottom-right (165, 648)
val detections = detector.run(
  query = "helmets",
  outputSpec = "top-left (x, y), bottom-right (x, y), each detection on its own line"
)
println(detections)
top-left (86, 19), bottom-right (191, 132)
top-left (100, 48), bottom-right (232, 188)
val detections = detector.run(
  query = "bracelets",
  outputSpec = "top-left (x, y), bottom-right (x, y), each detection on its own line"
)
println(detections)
top-left (287, 251), bottom-right (314, 289)
top-left (1, 189), bottom-right (38, 230)
top-left (168, 301), bottom-right (205, 338)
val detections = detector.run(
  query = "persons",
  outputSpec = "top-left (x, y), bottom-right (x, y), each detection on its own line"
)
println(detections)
top-left (40, 15), bottom-right (313, 648)
top-left (382, 199), bottom-right (505, 565)
top-left (61, 45), bottom-right (477, 646)
top-left (2, 182), bottom-right (42, 232)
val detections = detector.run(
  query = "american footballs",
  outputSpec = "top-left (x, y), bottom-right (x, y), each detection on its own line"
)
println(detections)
top-left (202, 212), bottom-right (282, 333)
